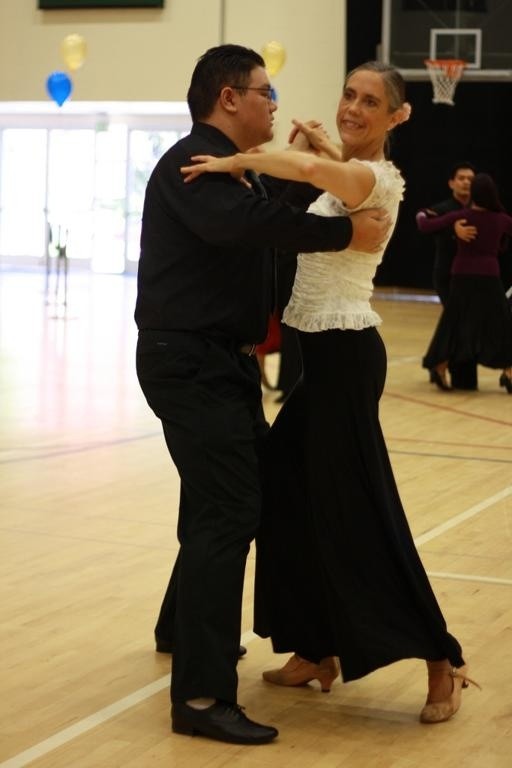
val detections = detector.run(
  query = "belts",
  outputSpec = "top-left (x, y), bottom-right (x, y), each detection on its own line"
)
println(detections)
top-left (233, 339), bottom-right (256, 357)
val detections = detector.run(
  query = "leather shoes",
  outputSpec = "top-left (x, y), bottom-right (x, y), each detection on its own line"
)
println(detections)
top-left (157, 638), bottom-right (247, 658)
top-left (172, 700), bottom-right (278, 744)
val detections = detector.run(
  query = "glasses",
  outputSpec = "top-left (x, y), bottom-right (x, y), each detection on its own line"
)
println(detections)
top-left (227, 84), bottom-right (276, 105)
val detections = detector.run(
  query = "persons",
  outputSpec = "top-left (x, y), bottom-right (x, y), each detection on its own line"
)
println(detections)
top-left (427, 164), bottom-right (492, 391)
top-left (258, 320), bottom-right (280, 394)
top-left (417, 174), bottom-right (510, 391)
top-left (136, 44), bottom-right (394, 747)
top-left (180, 58), bottom-right (468, 722)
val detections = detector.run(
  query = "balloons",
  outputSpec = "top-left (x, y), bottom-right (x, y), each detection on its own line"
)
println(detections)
top-left (262, 42), bottom-right (287, 74)
top-left (47, 73), bottom-right (72, 104)
top-left (60, 32), bottom-right (88, 68)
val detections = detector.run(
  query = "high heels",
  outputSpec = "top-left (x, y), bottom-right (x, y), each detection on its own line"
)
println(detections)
top-left (264, 658), bottom-right (338, 692)
top-left (499, 375), bottom-right (512, 393)
top-left (420, 664), bottom-right (469, 724)
top-left (426, 366), bottom-right (451, 390)
